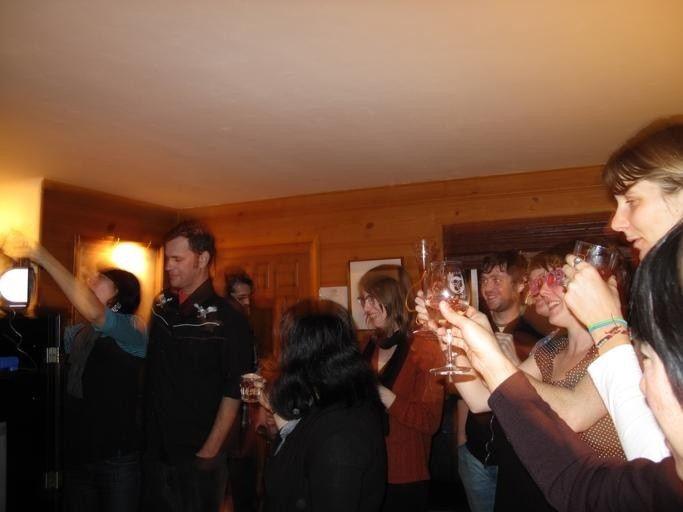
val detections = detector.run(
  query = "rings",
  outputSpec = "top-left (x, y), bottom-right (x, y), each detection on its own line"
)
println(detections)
top-left (572, 256), bottom-right (582, 264)
top-left (562, 277), bottom-right (572, 288)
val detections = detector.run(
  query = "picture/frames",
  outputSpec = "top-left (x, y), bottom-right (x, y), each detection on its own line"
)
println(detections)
top-left (345, 257), bottom-right (403, 332)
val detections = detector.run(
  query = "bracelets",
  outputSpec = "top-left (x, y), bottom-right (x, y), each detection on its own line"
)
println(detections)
top-left (582, 320), bottom-right (626, 335)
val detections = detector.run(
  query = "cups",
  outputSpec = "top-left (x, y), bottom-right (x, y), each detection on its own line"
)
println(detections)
top-left (573, 238), bottom-right (617, 282)
top-left (239, 375), bottom-right (264, 402)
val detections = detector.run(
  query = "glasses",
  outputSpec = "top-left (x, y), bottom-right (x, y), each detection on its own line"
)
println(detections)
top-left (357, 295), bottom-right (376, 306)
top-left (536, 270), bottom-right (564, 289)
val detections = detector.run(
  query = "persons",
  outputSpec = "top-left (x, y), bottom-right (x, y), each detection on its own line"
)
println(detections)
top-left (242, 297), bottom-right (392, 512)
top-left (136, 218), bottom-right (266, 512)
top-left (0, 235), bottom-right (147, 512)
top-left (415, 115), bottom-right (683, 512)
top-left (354, 263), bottom-right (443, 511)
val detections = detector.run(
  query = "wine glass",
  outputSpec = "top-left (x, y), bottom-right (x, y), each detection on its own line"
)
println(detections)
top-left (428, 258), bottom-right (473, 375)
top-left (416, 238), bottom-right (432, 295)
